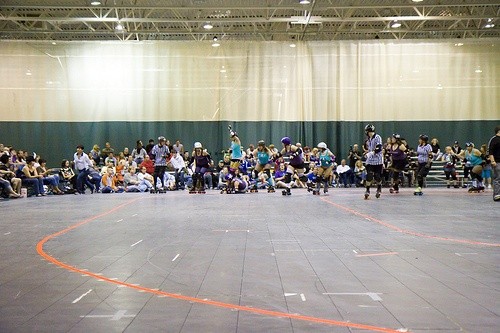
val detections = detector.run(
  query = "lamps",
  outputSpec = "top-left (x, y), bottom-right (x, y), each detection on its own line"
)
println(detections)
top-left (299, 0), bottom-right (311, 4)
top-left (391, 20), bottom-right (401, 28)
top-left (203, 21), bottom-right (213, 29)
top-left (211, 36), bottom-right (220, 46)
top-left (289, 37), bottom-right (296, 47)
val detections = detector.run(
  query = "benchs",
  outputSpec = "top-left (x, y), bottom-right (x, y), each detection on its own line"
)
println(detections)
top-left (410, 152), bottom-right (473, 187)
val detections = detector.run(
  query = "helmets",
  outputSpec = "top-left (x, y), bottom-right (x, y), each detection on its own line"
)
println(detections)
top-left (230, 132), bottom-right (239, 138)
top-left (317, 141), bottom-right (327, 148)
top-left (365, 123), bottom-right (429, 142)
top-left (194, 142), bottom-right (203, 149)
top-left (281, 137), bottom-right (291, 144)
top-left (158, 136), bottom-right (167, 142)
top-left (257, 140), bottom-right (265, 146)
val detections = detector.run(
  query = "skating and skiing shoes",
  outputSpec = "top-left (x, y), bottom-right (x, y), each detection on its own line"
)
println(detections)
top-left (220, 185), bottom-right (291, 195)
top-left (307, 180), bottom-right (313, 192)
top-left (468, 186), bottom-right (485, 193)
top-left (150, 188), bottom-right (157, 194)
top-left (364, 186), bottom-right (423, 199)
top-left (198, 187), bottom-right (206, 194)
top-left (189, 187), bottom-right (196, 194)
top-left (159, 187), bottom-right (166, 193)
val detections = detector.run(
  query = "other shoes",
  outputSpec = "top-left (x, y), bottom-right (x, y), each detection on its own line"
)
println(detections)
top-left (324, 189), bottom-right (329, 193)
top-left (494, 194), bottom-right (500, 201)
top-left (75, 192), bottom-right (82, 195)
top-left (91, 186), bottom-right (94, 194)
top-left (313, 189), bottom-right (320, 195)
top-left (9, 190), bottom-right (64, 198)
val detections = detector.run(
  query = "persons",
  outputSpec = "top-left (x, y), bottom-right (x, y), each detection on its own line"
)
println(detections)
top-left (413, 134), bottom-right (492, 196)
top-left (272, 137), bottom-right (312, 195)
top-left (150, 136), bottom-right (171, 194)
top-left (138, 166), bottom-right (154, 190)
top-left (362, 124), bottom-right (383, 199)
top-left (243, 141), bottom-right (277, 193)
top-left (187, 141), bottom-right (213, 194)
top-left (228, 125), bottom-right (246, 181)
top-left (0, 144), bottom-right (76, 199)
top-left (220, 173), bottom-right (258, 194)
top-left (489, 127), bottom-right (500, 200)
top-left (171, 139), bottom-right (228, 190)
top-left (389, 134), bottom-right (409, 194)
top-left (74, 144), bottom-right (96, 194)
top-left (89, 139), bottom-right (154, 193)
top-left (167, 150), bottom-right (186, 190)
top-left (312, 137), bottom-right (431, 193)
top-left (123, 166), bottom-right (147, 192)
top-left (98, 168), bottom-right (119, 193)
top-left (241, 142), bottom-right (329, 189)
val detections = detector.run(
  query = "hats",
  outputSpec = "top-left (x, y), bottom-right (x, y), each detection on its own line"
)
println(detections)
top-left (26, 156), bottom-right (35, 161)
top-left (454, 140), bottom-right (459, 144)
top-left (466, 141), bottom-right (473, 147)
top-left (0, 154), bottom-right (10, 163)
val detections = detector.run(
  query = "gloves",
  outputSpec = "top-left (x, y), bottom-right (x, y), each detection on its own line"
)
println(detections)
top-left (227, 125), bottom-right (233, 132)
